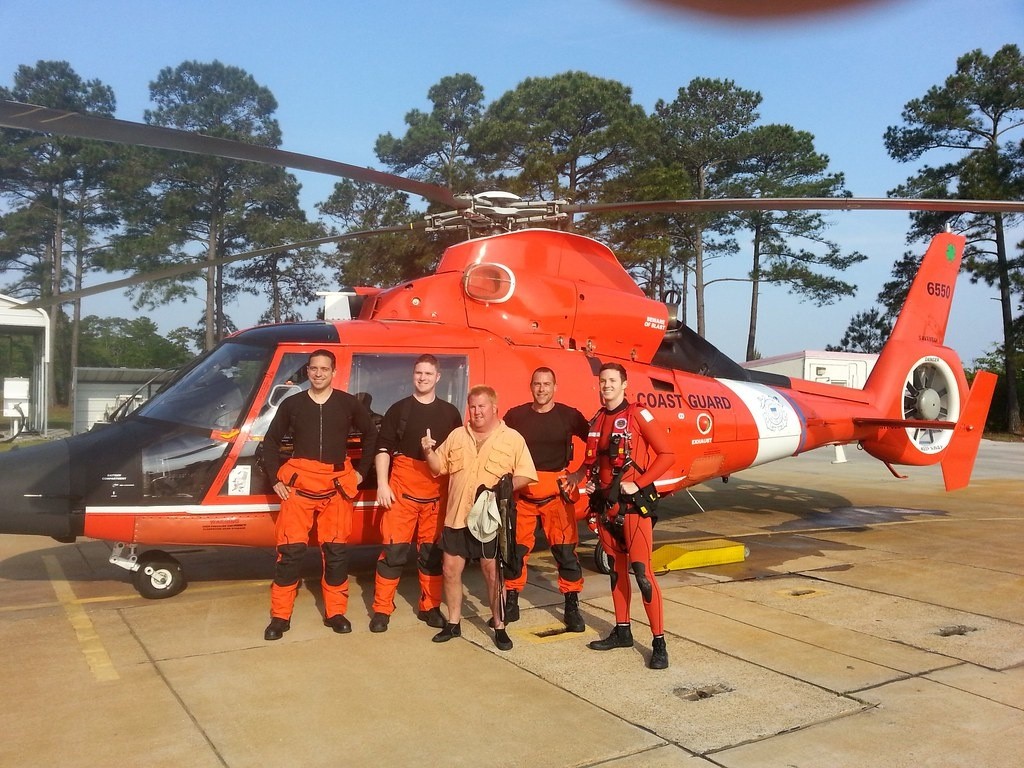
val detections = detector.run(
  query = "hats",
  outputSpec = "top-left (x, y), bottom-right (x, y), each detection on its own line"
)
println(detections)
top-left (467, 492), bottom-right (503, 542)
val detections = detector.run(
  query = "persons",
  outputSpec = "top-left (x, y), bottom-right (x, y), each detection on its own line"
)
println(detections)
top-left (263, 350), bottom-right (379, 640)
top-left (371, 355), bottom-right (463, 633)
top-left (420, 386), bottom-right (539, 651)
top-left (587, 363), bottom-right (676, 670)
top-left (488, 367), bottom-right (590, 632)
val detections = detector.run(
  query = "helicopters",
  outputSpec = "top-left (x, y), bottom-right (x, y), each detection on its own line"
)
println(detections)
top-left (0, 98), bottom-right (1024, 601)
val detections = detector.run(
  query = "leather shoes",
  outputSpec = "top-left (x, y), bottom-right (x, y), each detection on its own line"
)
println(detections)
top-left (418, 607), bottom-right (447, 628)
top-left (370, 611), bottom-right (389, 632)
top-left (324, 614), bottom-right (352, 633)
top-left (265, 617), bottom-right (291, 640)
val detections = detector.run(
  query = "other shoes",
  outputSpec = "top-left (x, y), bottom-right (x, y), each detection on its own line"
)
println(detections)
top-left (496, 630), bottom-right (513, 650)
top-left (432, 622), bottom-right (462, 642)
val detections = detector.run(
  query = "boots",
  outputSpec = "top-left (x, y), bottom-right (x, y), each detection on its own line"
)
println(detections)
top-left (489, 590), bottom-right (519, 628)
top-left (564, 591), bottom-right (586, 632)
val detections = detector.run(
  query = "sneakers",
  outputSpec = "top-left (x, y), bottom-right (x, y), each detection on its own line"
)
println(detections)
top-left (591, 625), bottom-right (633, 649)
top-left (650, 638), bottom-right (668, 668)
